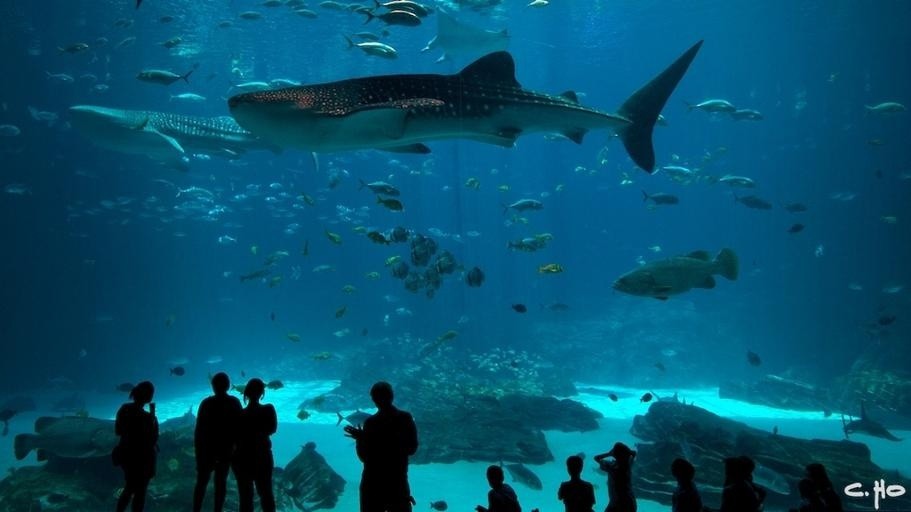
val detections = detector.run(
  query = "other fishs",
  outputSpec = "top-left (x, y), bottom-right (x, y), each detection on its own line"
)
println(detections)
top-left (0, 0), bottom-right (910, 512)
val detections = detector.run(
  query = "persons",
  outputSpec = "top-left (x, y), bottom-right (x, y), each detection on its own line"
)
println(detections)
top-left (712, 451), bottom-right (848, 512)
top-left (474, 464), bottom-right (521, 511)
top-left (229, 379), bottom-right (279, 511)
top-left (345, 382), bottom-right (419, 511)
top-left (560, 455), bottom-right (597, 511)
top-left (671, 456), bottom-right (707, 511)
top-left (595, 443), bottom-right (641, 512)
top-left (193, 370), bottom-right (230, 512)
top-left (114, 381), bottom-right (155, 512)
